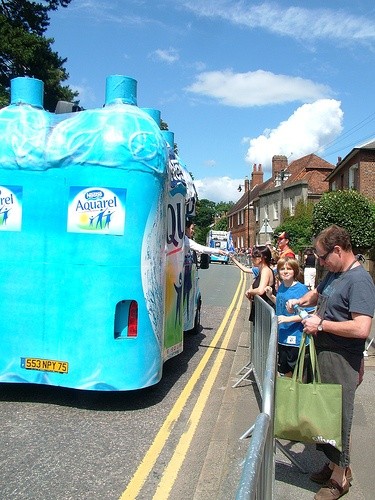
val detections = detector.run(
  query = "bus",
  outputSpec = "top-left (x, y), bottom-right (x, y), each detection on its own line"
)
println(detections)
top-left (205, 229), bottom-right (235, 265)
top-left (0, 74), bottom-right (212, 392)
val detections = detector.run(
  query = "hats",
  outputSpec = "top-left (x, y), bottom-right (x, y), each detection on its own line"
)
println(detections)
top-left (273, 232), bottom-right (289, 239)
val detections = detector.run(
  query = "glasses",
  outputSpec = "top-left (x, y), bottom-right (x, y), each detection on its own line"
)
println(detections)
top-left (251, 255), bottom-right (260, 259)
top-left (318, 248), bottom-right (334, 261)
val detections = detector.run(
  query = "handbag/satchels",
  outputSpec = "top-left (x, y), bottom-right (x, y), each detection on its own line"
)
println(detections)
top-left (274, 331), bottom-right (342, 452)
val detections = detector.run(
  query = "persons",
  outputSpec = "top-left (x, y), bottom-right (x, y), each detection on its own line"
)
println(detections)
top-left (270, 231), bottom-right (295, 261)
top-left (184, 220), bottom-right (227, 257)
top-left (229, 245), bottom-right (252, 257)
top-left (264, 256), bottom-right (315, 378)
top-left (297, 250), bottom-right (320, 291)
top-left (285, 224), bottom-right (375, 500)
top-left (244, 246), bottom-right (277, 326)
top-left (228, 253), bottom-right (279, 284)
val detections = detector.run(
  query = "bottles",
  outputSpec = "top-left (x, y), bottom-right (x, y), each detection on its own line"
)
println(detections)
top-left (294, 304), bottom-right (309, 321)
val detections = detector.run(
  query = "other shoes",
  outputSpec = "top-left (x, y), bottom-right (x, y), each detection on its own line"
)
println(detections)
top-left (310, 463), bottom-right (353, 483)
top-left (313, 479), bottom-right (349, 500)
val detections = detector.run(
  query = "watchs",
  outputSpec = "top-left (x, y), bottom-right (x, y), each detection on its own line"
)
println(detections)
top-left (317, 319), bottom-right (323, 331)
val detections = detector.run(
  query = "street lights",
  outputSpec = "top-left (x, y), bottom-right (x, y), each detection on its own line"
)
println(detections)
top-left (237, 180), bottom-right (250, 248)
